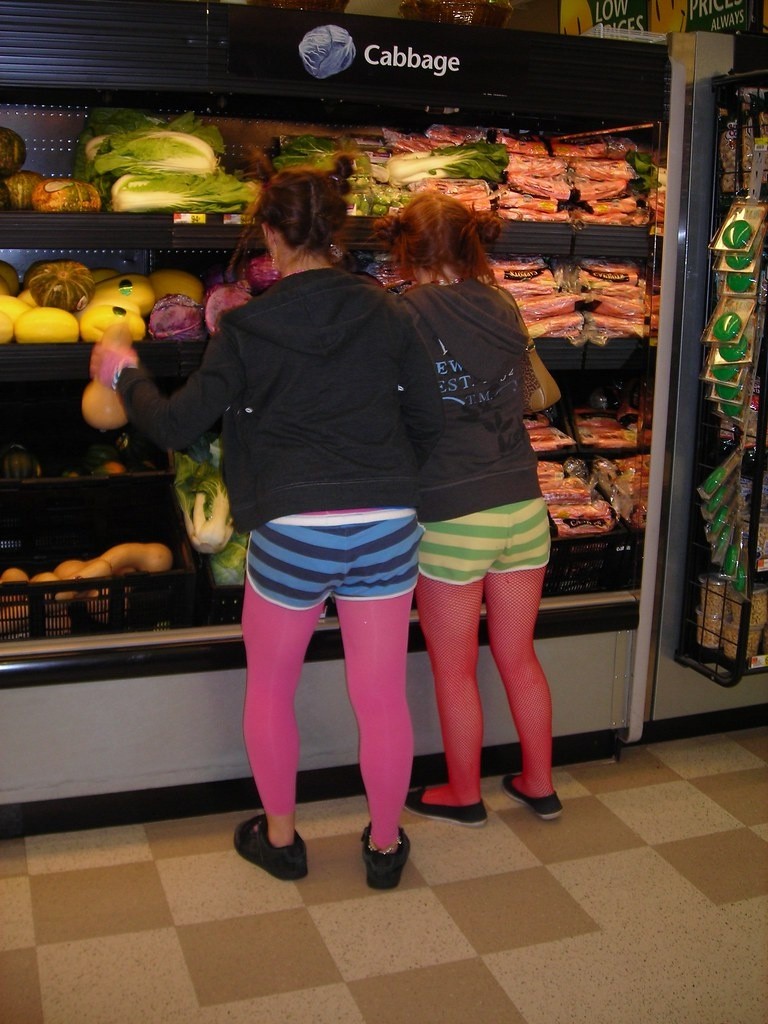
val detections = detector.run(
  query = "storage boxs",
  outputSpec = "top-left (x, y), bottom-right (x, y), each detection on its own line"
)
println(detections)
top-left (543, 493), bottom-right (632, 597)
top-left (568, 402), bottom-right (635, 460)
top-left (0, 391), bottom-right (175, 501)
top-left (1, 507), bottom-right (244, 644)
top-left (523, 399), bottom-right (576, 460)
top-left (597, 483), bottom-right (645, 591)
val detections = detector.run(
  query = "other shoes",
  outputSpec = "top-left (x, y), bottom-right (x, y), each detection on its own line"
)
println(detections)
top-left (233, 814), bottom-right (308, 881)
top-left (361, 821), bottom-right (411, 891)
top-left (502, 773), bottom-right (563, 820)
top-left (404, 787), bottom-right (488, 827)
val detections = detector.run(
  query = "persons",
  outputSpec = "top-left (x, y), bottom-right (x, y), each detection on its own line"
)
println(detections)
top-left (360, 190), bottom-right (563, 826)
top-left (89, 154), bottom-right (445, 889)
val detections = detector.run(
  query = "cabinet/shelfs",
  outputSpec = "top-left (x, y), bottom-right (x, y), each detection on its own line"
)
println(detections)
top-left (1, 0), bottom-right (686, 834)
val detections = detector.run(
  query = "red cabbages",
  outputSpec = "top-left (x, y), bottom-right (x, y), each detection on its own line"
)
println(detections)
top-left (148, 254), bottom-right (285, 344)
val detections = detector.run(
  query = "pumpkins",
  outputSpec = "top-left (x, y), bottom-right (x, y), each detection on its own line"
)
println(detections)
top-left (0, 435), bottom-right (144, 477)
top-left (0, 125), bottom-right (102, 213)
top-left (0, 252), bottom-right (205, 344)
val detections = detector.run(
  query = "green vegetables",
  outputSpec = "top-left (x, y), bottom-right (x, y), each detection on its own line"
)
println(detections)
top-left (631, 149), bottom-right (658, 192)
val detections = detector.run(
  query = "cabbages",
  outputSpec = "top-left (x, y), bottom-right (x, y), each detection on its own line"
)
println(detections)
top-left (76, 110), bottom-right (508, 213)
top-left (173, 454), bottom-right (248, 586)
